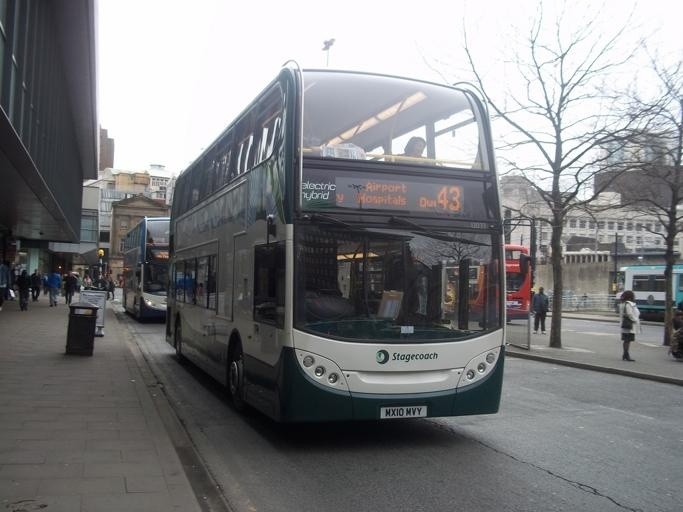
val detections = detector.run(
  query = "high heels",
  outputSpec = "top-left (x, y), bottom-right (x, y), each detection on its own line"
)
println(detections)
top-left (622, 350), bottom-right (636, 362)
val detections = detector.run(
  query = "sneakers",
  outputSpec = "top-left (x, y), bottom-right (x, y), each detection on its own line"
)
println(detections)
top-left (533, 330), bottom-right (538, 335)
top-left (541, 330), bottom-right (549, 334)
top-left (49, 301), bottom-right (58, 309)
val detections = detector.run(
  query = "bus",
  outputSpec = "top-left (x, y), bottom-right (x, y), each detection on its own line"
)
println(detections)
top-left (166, 59), bottom-right (508, 439)
top-left (505, 245), bottom-right (531, 323)
top-left (614, 265), bottom-right (682, 317)
top-left (122, 217), bottom-right (170, 323)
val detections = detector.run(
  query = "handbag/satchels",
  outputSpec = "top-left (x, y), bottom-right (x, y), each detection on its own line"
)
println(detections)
top-left (620, 314), bottom-right (633, 330)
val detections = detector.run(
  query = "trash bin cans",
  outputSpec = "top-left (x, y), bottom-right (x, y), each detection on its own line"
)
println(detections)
top-left (66, 302), bottom-right (99, 356)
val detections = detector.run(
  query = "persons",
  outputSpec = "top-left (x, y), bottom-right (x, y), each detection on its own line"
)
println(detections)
top-left (619, 290), bottom-right (641, 361)
top-left (531, 288), bottom-right (548, 334)
top-left (0, 256), bottom-right (114, 312)
